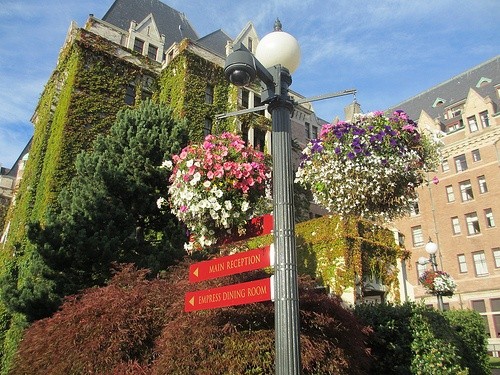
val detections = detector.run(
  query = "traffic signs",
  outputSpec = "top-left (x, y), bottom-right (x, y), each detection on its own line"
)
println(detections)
top-left (189, 245), bottom-right (273, 284)
top-left (190, 214), bottom-right (273, 253)
top-left (183, 277), bottom-right (276, 313)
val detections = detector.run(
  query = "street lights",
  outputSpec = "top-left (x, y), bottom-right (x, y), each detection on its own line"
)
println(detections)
top-left (223, 16), bottom-right (303, 375)
top-left (416, 235), bottom-right (445, 313)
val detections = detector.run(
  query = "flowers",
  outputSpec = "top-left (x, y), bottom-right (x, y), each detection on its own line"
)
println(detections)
top-left (156, 132), bottom-right (273, 256)
top-left (293, 110), bottom-right (451, 224)
top-left (418, 270), bottom-right (456, 300)
